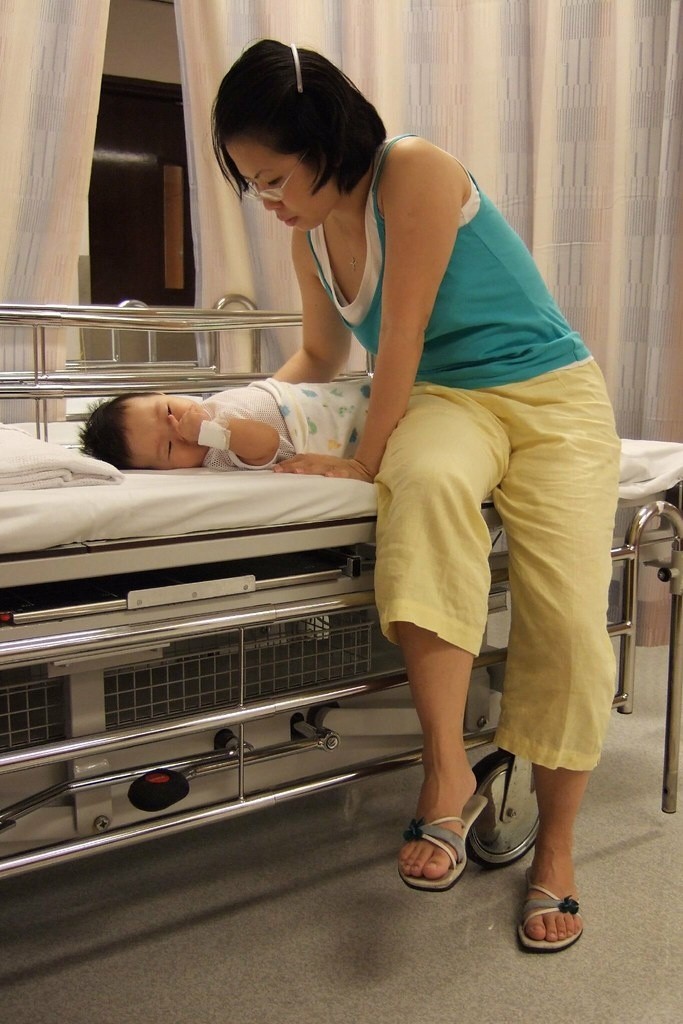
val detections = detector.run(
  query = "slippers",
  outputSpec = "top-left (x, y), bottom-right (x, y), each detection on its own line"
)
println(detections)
top-left (517, 869), bottom-right (584, 953)
top-left (396, 795), bottom-right (489, 888)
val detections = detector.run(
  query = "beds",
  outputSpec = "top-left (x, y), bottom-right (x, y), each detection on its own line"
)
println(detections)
top-left (0, 292), bottom-right (683, 883)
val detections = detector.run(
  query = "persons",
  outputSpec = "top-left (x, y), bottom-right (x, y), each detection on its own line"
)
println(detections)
top-left (80, 378), bottom-right (373, 470)
top-left (212, 38), bottom-right (619, 952)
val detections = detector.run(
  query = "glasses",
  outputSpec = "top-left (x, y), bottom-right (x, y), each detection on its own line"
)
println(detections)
top-left (246, 148), bottom-right (306, 201)
